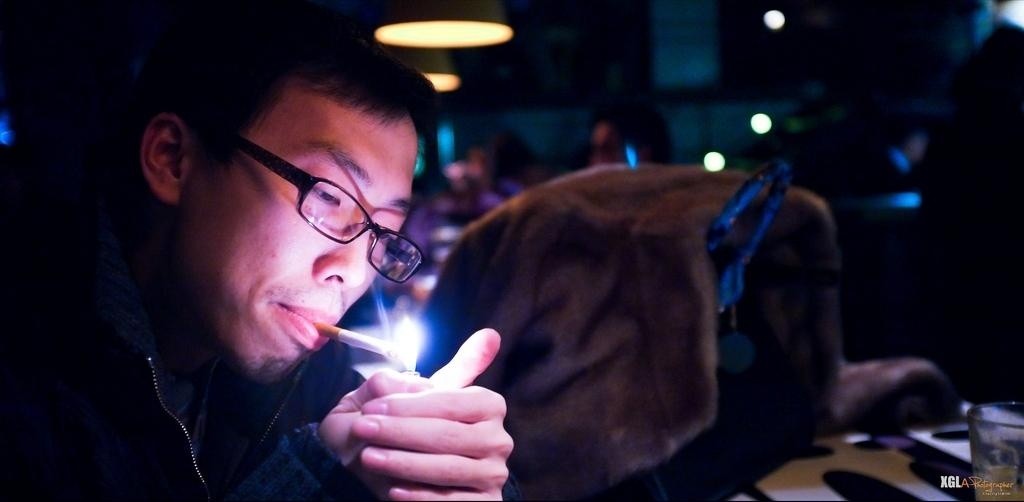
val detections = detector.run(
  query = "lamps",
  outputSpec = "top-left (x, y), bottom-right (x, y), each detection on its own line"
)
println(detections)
top-left (370, 1), bottom-right (515, 95)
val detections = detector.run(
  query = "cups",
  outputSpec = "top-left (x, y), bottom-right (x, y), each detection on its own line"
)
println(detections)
top-left (968, 401), bottom-right (1022, 499)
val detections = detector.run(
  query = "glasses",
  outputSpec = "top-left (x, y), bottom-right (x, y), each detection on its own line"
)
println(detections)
top-left (233, 133), bottom-right (424, 284)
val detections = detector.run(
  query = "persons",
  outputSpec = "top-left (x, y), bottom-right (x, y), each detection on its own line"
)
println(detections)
top-left (1, 0), bottom-right (1024, 502)
